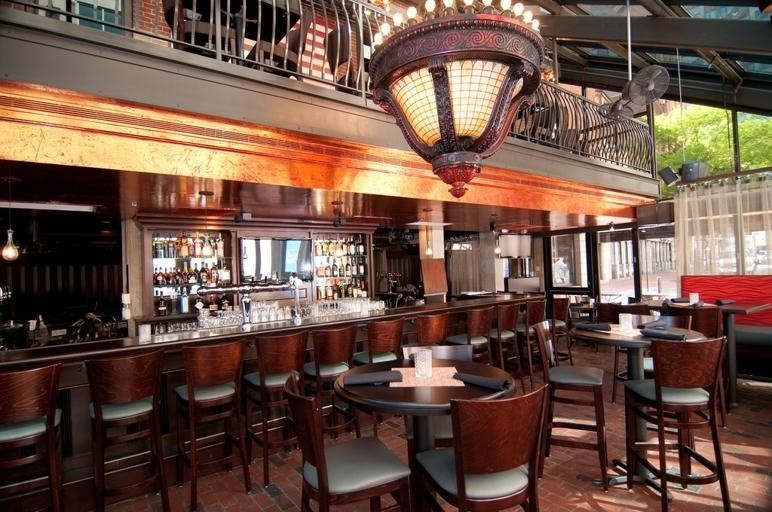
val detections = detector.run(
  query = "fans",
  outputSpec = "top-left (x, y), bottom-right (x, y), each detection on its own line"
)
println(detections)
top-left (625, 64), bottom-right (671, 105)
top-left (598, 102), bottom-right (636, 122)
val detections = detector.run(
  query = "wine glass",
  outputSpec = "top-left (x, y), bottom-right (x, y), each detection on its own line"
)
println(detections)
top-left (197, 297), bottom-right (386, 329)
top-left (153, 321), bottom-right (197, 336)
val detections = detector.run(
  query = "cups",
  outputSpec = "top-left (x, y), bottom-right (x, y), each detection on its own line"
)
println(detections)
top-left (619, 313), bottom-right (632, 332)
top-left (137, 324), bottom-right (152, 344)
top-left (414, 349), bottom-right (433, 379)
top-left (689, 292), bottom-right (700, 305)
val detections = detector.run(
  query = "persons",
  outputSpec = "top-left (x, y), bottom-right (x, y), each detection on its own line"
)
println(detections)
top-left (553, 257), bottom-right (566, 283)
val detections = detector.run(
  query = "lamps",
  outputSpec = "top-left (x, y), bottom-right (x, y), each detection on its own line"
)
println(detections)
top-left (2, 203), bottom-right (20, 261)
top-left (658, 161), bottom-right (707, 186)
top-left (367, 0), bottom-right (545, 198)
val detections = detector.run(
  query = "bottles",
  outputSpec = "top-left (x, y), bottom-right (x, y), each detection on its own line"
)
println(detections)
top-left (151, 231), bottom-right (231, 317)
top-left (38, 314), bottom-right (49, 344)
top-left (314, 234), bottom-right (369, 301)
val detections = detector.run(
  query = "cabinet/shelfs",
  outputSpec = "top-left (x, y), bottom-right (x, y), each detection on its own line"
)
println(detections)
top-left (132, 211), bottom-right (237, 318)
top-left (310, 216), bottom-right (384, 300)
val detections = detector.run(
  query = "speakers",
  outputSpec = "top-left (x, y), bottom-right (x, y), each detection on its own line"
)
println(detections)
top-left (658, 166), bottom-right (679, 187)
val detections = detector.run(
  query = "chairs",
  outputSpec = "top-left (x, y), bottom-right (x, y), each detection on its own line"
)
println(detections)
top-left (161, 0), bottom-right (377, 96)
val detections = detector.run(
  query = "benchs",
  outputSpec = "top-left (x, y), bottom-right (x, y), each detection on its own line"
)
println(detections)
top-left (681, 275), bottom-right (772, 344)
